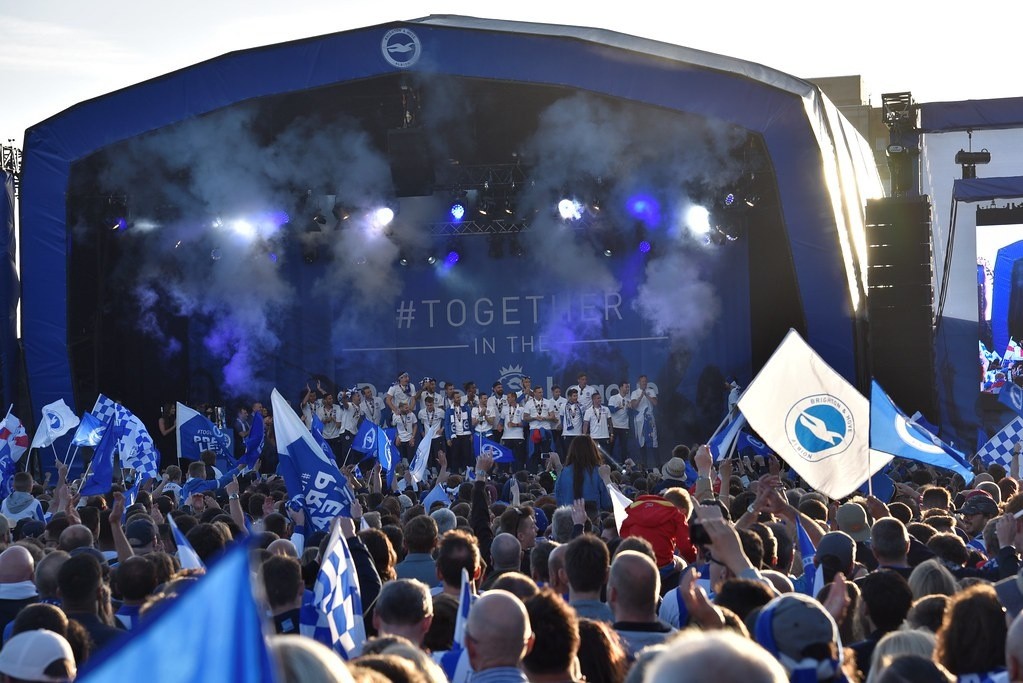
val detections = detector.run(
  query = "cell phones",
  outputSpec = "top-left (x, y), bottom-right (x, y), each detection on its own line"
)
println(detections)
top-left (540, 453), bottom-right (550, 459)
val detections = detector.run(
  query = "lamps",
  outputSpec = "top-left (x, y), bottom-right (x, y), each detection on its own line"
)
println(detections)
top-left (167, 237), bottom-right (185, 251)
top-left (298, 191), bottom-right (550, 267)
top-left (955, 149), bottom-right (991, 179)
top-left (99, 209), bottom-right (123, 230)
top-left (587, 180), bottom-right (761, 259)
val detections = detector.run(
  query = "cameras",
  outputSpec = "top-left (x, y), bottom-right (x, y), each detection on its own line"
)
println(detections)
top-left (731, 459), bottom-right (739, 464)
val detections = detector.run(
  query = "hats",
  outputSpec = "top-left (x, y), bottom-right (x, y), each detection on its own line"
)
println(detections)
top-left (953, 494), bottom-right (999, 518)
top-left (745, 592), bottom-right (837, 659)
top-left (486, 485), bottom-right (498, 504)
top-left (0, 628), bottom-right (77, 682)
top-left (835, 502), bottom-right (871, 542)
top-left (69, 546), bottom-right (111, 581)
top-left (662, 456), bottom-right (688, 481)
top-left (532, 507), bottom-right (550, 531)
top-left (816, 531), bottom-right (857, 570)
top-left (125, 518), bottom-right (159, 548)
top-left (964, 489), bottom-right (993, 501)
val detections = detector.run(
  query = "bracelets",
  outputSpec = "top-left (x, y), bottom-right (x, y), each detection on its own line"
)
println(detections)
top-left (1012, 451), bottom-right (1019, 455)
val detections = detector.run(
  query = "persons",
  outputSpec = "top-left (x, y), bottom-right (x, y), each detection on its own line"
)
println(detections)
top-left (725, 376), bottom-right (741, 424)
top-left (0, 436), bottom-right (1023, 683)
top-left (979, 336), bottom-right (1023, 394)
top-left (156, 371), bottom-right (661, 468)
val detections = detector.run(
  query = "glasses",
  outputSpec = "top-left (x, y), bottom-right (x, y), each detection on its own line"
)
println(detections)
top-left (704, 551), bottom-right (726, 567)
top-left (512, 506), bottom-right (541, 538)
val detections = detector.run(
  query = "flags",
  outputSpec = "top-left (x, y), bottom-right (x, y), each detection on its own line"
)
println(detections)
top-left (428, 568), bottom-right (472, 683)
top-left (870, 376), bottom-right (975, 487)
top-left (377, 426), bottom-right (400, 490)
top-left (352, 419), bottom-right (398, 458)
top-left (473, 433), bottom-right (516, 463)
top-left (395, 425), bottom-right (434, 492)
top-left (270, 387), bottom-right (355, 533)
top-left (230, 412), bottom-right (265, 479)
top-left (0, 393), bottom-right (160, 524)
top-left (314, 524), bottom-right (367, 660)
top-left (738, 327), bottom-right (894, 500)
top-left (979, 416), bottom-right (1023, 479)
top-left (176, 401), bottom-right (227, 459)
top-left (73, 536), bottom-right (282, 683)
top-left (168, 513), bottom-right (206, 569)
